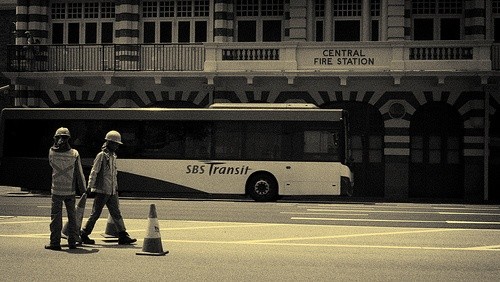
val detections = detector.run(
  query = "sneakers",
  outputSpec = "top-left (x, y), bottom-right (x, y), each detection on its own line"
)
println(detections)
top-left (68, 243), bottom-right (77, 251)
top-left (118, 235), bottom-right (137, 246)
top-left (80, 231), bottom-right (96, 245)
top-left (44, 242), bottom-right (61, 250)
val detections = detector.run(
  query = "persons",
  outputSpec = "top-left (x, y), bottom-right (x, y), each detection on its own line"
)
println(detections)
top-left (79, 131), bottom-right (137, 245)
top-left (44, 127), bottom-right (88, 250)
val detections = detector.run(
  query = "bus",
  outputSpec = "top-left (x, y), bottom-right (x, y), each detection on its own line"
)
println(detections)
top-left (0, 103), bottom-right (355, 202)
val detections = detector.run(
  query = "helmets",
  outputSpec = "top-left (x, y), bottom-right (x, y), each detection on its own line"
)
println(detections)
top-left (54, 127), bottom-right (71, 137)
top-left (104, 130), bottom-right (124, 145)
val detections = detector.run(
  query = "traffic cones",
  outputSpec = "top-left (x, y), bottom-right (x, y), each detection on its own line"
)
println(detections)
top-left (61, 193), bottom-right (87, 245)
top-left (101, 213), bottom-right (129, 239)
top-left (136, 204), bottom-right (169, 256)
